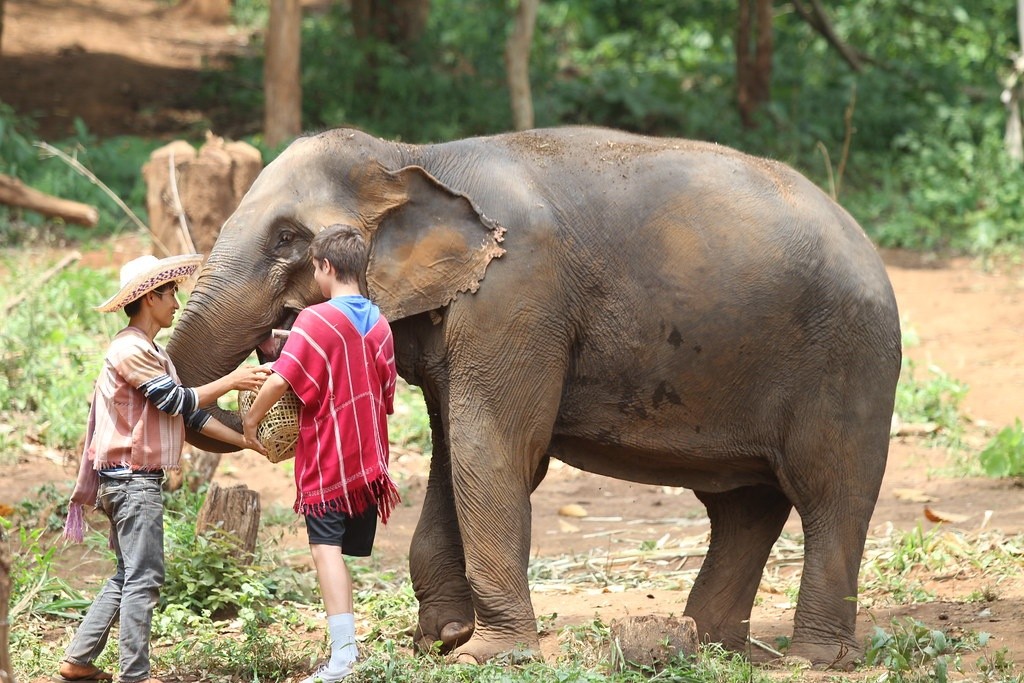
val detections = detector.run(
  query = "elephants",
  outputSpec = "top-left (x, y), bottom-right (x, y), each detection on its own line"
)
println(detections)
top-left (167, 124), bottom-right (900, 668)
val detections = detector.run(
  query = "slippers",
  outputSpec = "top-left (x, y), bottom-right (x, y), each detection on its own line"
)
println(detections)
top-left (52, 669), bottom-right (113, 683)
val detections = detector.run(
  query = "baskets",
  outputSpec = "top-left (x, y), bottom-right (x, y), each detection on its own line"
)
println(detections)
top-left (238, 362), bottom-right (302, 464)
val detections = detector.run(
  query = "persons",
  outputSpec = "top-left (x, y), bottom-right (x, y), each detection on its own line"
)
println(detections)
top-left (61, 254), bottom-right (273, 683)
top-left (242, 224), bottom-right (401, 683)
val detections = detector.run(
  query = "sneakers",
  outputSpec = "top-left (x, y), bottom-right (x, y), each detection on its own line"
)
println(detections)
top-left (299, 652), bottom-right (360, 683)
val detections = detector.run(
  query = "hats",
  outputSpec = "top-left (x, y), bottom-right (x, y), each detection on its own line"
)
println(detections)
top-left (92, 254), bottom-right (204, 313)
